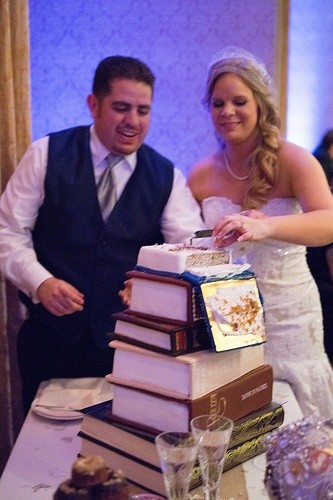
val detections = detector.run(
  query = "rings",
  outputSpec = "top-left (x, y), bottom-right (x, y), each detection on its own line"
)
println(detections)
top-left (239, 220), bottom-right (245, 229)
top-left (247, 233), bottom-right (253, 242)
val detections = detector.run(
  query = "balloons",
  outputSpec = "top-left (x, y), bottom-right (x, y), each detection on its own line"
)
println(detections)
top-left (263, 416), bottom-right (333, 500)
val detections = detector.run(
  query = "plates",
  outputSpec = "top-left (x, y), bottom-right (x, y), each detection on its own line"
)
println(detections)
top-left (32, 398), bottom-right (84, 420)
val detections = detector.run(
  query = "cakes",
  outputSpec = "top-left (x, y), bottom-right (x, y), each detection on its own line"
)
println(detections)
top-left (136, 241), bottom-right (232, 275)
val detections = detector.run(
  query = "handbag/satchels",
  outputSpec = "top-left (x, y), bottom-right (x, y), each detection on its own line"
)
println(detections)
top-left (265, 419), bottom-right (333, 500)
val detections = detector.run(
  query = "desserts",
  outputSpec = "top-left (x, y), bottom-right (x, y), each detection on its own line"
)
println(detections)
top-left (53, 453), bottom-right (130, 500)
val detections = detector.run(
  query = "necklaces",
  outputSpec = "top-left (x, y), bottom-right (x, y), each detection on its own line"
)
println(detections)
top-left (223, 149), bottom-right (256, 180)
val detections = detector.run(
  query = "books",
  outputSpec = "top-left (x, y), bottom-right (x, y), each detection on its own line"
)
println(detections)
top-left (71, 272), bottom-right (285, 500)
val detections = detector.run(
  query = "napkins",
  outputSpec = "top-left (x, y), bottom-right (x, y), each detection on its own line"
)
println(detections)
top-left (36, 377), bottom-right (114, 411)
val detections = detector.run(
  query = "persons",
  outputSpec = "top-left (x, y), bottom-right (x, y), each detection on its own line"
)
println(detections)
top-left (0, 55), bottom-right (203, 419)
top-left (185, 53), bottom-right (333, 428)
top-left (307, 126), bottom-right (333, 367)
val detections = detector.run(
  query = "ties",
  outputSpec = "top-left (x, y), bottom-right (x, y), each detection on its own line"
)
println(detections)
top-left (98, 154), bottom-right (124, 222)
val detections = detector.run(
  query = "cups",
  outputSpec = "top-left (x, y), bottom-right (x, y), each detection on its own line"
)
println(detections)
top-left (155, 431), bottom-right (198, 500)
top-left (190, 414), bottom-right (234, 499)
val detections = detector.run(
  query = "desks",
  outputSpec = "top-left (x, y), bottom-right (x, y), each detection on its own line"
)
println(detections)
top-left (0, 372), bottom-right (305, 500)
